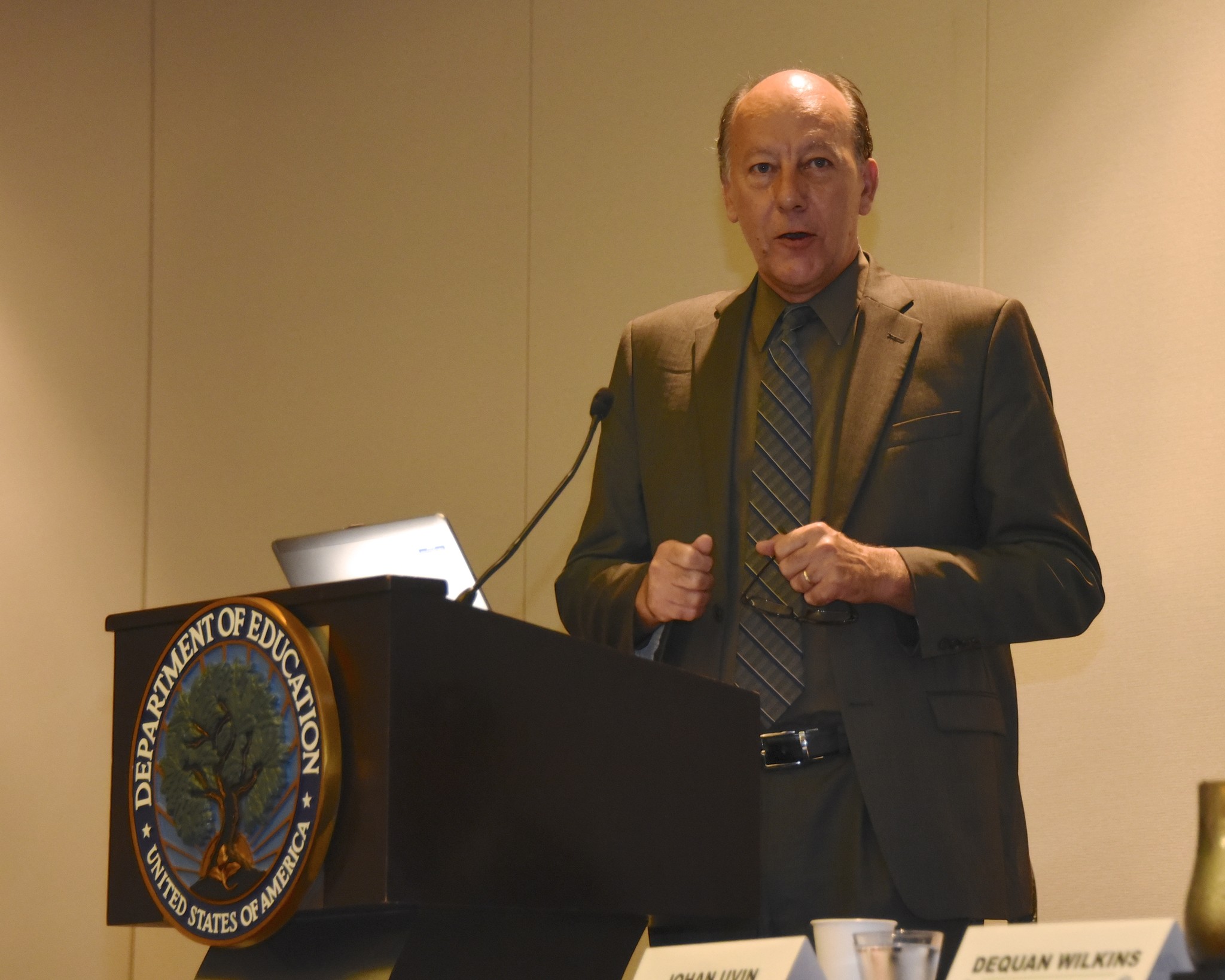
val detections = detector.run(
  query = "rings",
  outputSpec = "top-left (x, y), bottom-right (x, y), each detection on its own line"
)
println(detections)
top-left (804, 569), bottom-right (813, 583)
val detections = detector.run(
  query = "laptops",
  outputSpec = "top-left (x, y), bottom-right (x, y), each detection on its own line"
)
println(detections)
top-left (271, 514), bottom-right (492, 612)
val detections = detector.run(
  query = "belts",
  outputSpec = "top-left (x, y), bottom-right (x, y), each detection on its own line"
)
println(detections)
top-left (759, 725), bottom-right (849, 768)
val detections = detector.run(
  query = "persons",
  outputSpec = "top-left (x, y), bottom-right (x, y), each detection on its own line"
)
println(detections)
top-left (554, 67), bottom-right (1103, 980)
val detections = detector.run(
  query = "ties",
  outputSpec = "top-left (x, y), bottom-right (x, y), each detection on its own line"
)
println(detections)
top-left (733, 305), bottom-right (813, 726)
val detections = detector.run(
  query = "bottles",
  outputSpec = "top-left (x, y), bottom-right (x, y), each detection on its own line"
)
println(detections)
top-left (1184, 779), bottom-right (1225, 980)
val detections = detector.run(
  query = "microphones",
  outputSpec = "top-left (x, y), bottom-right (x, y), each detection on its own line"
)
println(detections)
top-left (454, 387), bottom-right (616, 605)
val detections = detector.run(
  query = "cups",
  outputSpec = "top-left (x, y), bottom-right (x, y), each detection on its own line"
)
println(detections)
top-left (852, 929), bottom-right (944, 980)
top-left (809, 919), bottom-right (899, 980)
